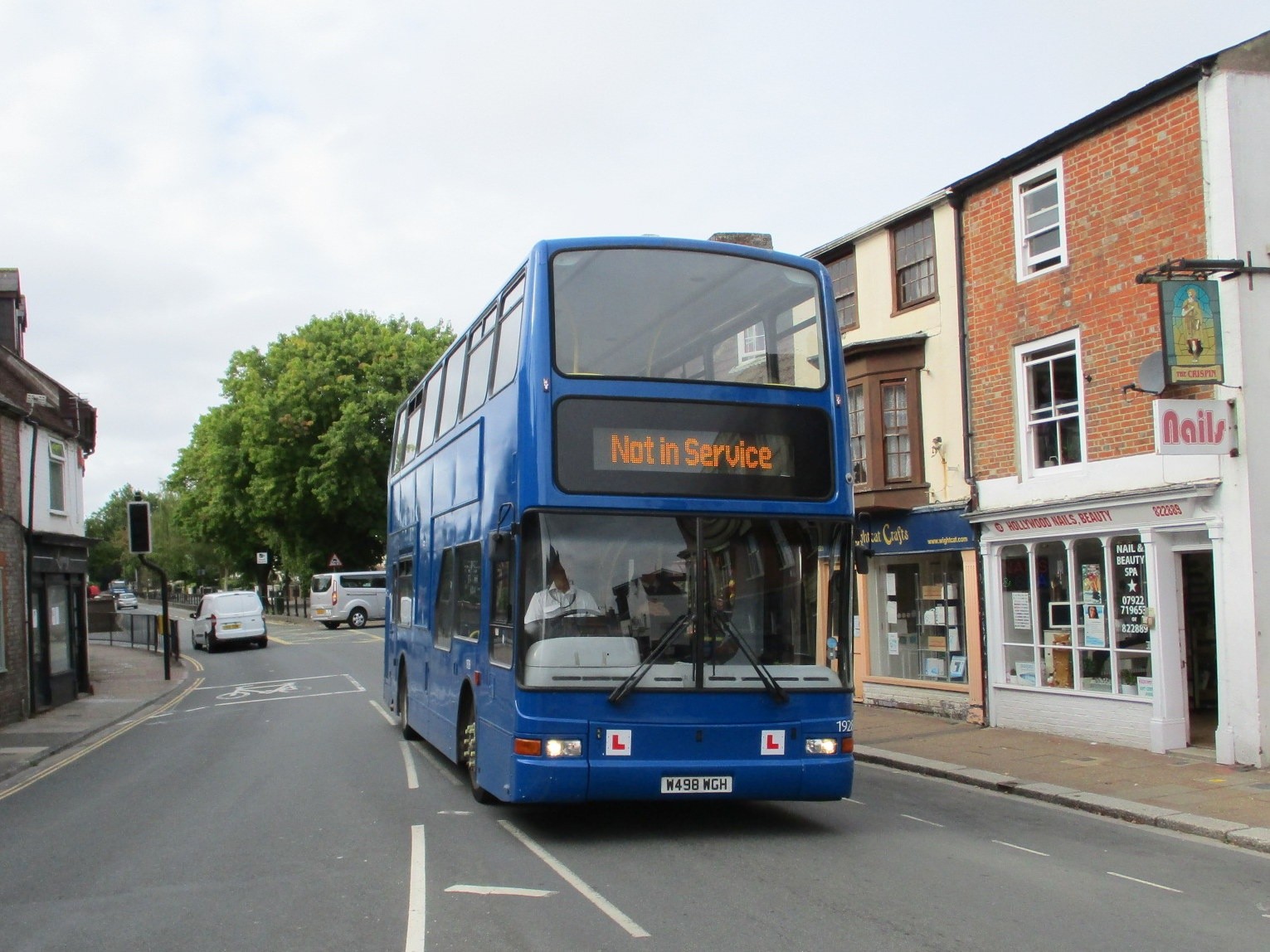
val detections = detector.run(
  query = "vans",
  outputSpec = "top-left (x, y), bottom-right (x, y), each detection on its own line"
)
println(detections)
top-left (310, 570), bottom-right (387, 629)
top-left (189, 591), bottom-right (269, 652)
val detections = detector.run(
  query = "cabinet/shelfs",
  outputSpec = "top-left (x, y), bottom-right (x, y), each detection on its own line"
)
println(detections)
top-left (915, 572), bottom-right (968, 682)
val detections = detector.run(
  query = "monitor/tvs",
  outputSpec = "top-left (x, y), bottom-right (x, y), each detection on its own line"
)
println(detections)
top-left (1049, 602), bottom-right (1085, 630)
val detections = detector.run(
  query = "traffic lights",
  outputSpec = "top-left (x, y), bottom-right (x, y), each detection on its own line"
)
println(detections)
top-left (127, 500), bottom-right (153, 556)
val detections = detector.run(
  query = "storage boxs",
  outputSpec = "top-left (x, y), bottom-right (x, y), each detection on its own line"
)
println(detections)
top-left (922, 582), bottom-right (961, 675)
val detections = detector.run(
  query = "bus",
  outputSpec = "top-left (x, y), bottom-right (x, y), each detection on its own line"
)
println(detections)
top-left (110, 581), bottom-right (126, 597)
top-left (369, 237), bottom-right (875, 806)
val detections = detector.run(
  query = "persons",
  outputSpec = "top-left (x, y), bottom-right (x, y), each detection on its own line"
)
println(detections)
top-left (722, 579), bottom-right (737, 621)
top-left (524, 553), bottom-right (603, 642)
top-left (1083, 573), bottom-right (1101, 600)
top-left (1088, 606), bottom-right (1099, 619)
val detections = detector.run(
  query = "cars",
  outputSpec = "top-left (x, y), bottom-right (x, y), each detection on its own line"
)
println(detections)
top-left (116, 593), bottom-right (138, 609)
